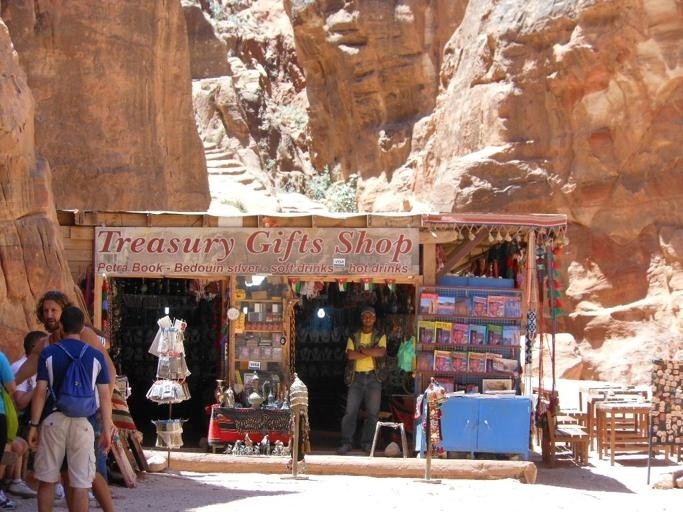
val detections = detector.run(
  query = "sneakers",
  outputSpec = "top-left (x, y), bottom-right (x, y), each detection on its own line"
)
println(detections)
top-left (56, 486), bottom-right (66, 498)
top-left (9, 480), bottom-right (38, 496)
top-left (0, 490), bottom-right (16, 509)
top-left (336, 443), bottom-right (372, 454)
top-left (89, 490), bottom-right (95, 501)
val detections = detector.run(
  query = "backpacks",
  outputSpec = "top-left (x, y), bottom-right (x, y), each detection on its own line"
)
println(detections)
top-left (49, 342), bottom-right (96, 417)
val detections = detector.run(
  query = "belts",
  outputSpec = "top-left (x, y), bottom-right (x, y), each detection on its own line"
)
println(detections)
top-left (355, 370), bottom-right (375, 375)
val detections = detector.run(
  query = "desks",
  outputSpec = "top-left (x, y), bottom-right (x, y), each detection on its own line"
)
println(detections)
top-left (210, 405), bottom-right (295, 453)
top-left (579, 387), bottom-right (669, 466)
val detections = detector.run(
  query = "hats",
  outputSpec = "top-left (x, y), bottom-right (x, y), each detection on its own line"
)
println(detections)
top-left (361, 307), bottom-right (374, 316)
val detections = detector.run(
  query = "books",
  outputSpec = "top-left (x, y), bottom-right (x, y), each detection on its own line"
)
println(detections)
top-left (416, 293), bottom-right (531, 399)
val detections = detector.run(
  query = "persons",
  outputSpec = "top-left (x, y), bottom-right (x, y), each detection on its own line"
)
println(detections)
top-left (0, 290), bottom-right (116, 512)
top-left (333, 305), bottom-right (388, 457)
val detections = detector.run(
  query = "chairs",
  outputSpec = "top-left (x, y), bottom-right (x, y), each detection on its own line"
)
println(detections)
top-left (530, 387), bottom-right (590, 469)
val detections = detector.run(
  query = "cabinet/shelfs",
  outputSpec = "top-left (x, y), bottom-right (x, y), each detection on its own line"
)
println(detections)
top-left (414, 396), bottom-right (531, 461)
top-left (229, 276), bottom-right (290, 390)
top-left (415, 286), bottom-right (523, 396)
